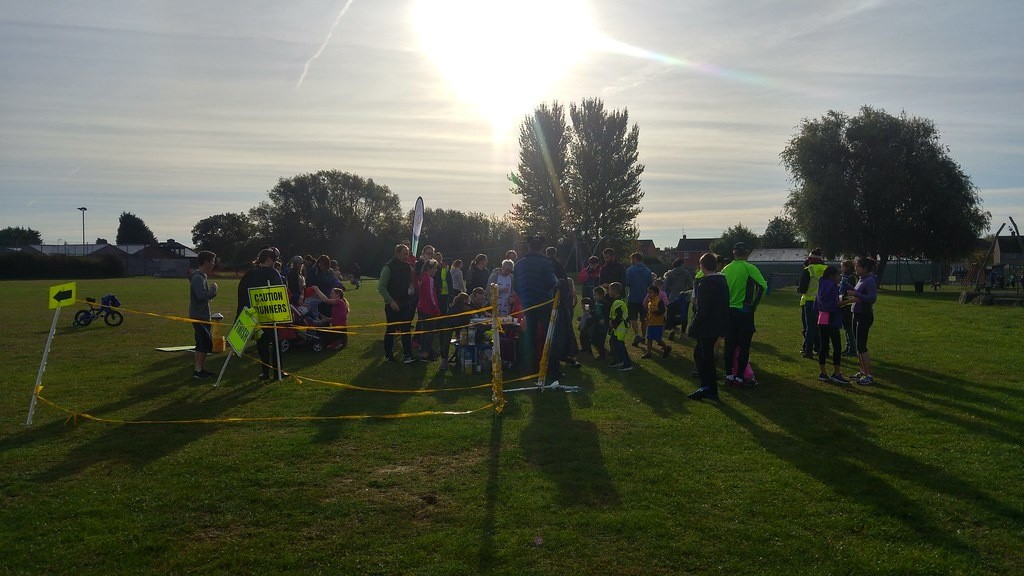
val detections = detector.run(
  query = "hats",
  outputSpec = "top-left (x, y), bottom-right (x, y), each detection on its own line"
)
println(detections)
top-left (734, 242), bottom-right (749, 250)
top-left (808, 247), bottom-right (822, 259)
top-left (305, 286), bottom-right (315, 297)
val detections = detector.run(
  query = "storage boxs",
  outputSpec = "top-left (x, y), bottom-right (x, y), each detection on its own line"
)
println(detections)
top-left (457, 343), bottom-right (493, 367)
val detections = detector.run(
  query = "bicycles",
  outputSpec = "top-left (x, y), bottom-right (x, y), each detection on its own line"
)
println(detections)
top-left (73, 296), bottom-right (125, 328)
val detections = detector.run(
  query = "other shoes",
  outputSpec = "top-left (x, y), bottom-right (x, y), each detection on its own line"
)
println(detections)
top-left (841, 349), bottom-right (857, 356)
top-left (440, 360), bottom-right (447, 369)
top-left (680, 334), bottom-right (687, 339)
top-left (274, 373), bottom-right (290, 380)
top-left (573, 361), bottom-right (581, 368)
top-left (800, 350), bottom-right (813, 360)
top-left (641, 353), bottom-right (650, 358)
top-left (664, 346), bottom-right (672, 357)
top-left (669, 331), bottom-right (675, 340)
top-left (259, 374), bottom-right (268, 379)
top-left (555, 374), bottom-right (566, 379)
top-left (421, 357), bottom-right (431, 363)
top-left (632, 336), bottom-right (641, 345)
top-left (327, 342), bottom-right (342, 349)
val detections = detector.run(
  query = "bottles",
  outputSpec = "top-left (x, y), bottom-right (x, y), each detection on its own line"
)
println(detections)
top-left (468, 322), bottom-right (477, 345)
top-left (460, 327), bottom-right (467, 344)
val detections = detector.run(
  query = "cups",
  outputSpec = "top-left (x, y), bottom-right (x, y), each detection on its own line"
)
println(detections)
top-left (513, 318), bottom-right (518, 323)
top-left (585, 304), bottom-right (589, 310)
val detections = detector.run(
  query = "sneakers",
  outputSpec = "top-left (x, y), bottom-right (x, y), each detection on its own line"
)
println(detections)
top-left (819, 373), bottom-right (830, 380)
top-left (608, 361), bottom-right (623, 368)
top-left (688, 386), bottom-right (717, 400)
top-left (726, 374), bottom-right (734, 384)
top-left (733, 376), bottom-right (757, 387)
top-left (404, 356), bottom-right (417, 364)
top-left (385, 355), bottom-right (395, 363)
top-left (830, 373), bottom-right (849, 383)
top-left (857, 375), bottom-right (873, 385)
top-left (194, 370), bottom-right (211, 379)
top-left (616, 363), bottom-right (633, 370)
top-left (850, 371), bottom-right (862, 379)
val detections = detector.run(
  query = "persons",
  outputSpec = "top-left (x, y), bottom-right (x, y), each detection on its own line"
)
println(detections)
top-left (352, 263), bottom-right (362, 289)
top-left (378, 237), bottom-right (767, 400)
top-left (188, 250), bottom-right (218, 380)
top-left (238, 246), bottom-right (349, 379)
top-left (990, 270), bottom-right (1024, 290)
top-left (795, 248), bottom-right (879, 385)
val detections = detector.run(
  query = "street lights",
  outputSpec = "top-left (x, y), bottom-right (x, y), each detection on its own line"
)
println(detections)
top-left (77, 206), bottom-right (87, 257)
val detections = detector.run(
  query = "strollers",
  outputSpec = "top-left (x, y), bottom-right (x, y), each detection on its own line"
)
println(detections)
top-left (276, 304), bottom-right (334, 352)
